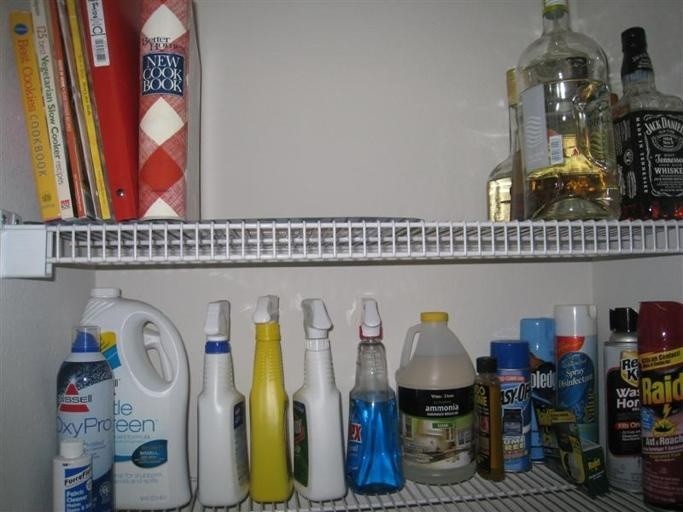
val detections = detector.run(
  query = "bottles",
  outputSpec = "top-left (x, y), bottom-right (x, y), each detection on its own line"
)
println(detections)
top-left (518, 319), bottom-right (556, 461)
top-left (613, 29), bottom-right (683, 219)
top-left (474, 355), bottom-right (506, 482)
top-left (52, 441), bottom-right (90, 512)
top-left (516, 33), bottom-right (619, 219)
top-left (492, 340), bottom-right (531, 471)
top-left (487, 70), bottom-right (515, 221)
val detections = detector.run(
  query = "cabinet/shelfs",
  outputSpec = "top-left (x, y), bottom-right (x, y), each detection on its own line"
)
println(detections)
top-left (0, 208), bottom-right (683, 511)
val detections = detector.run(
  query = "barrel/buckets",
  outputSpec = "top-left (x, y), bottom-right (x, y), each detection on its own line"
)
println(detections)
top-left (81, 288), bottom-right (191, 510)
top-left (395, 312), bottom-right (477, 483)
top-left (144, 328), bottom-right (173, 382)
